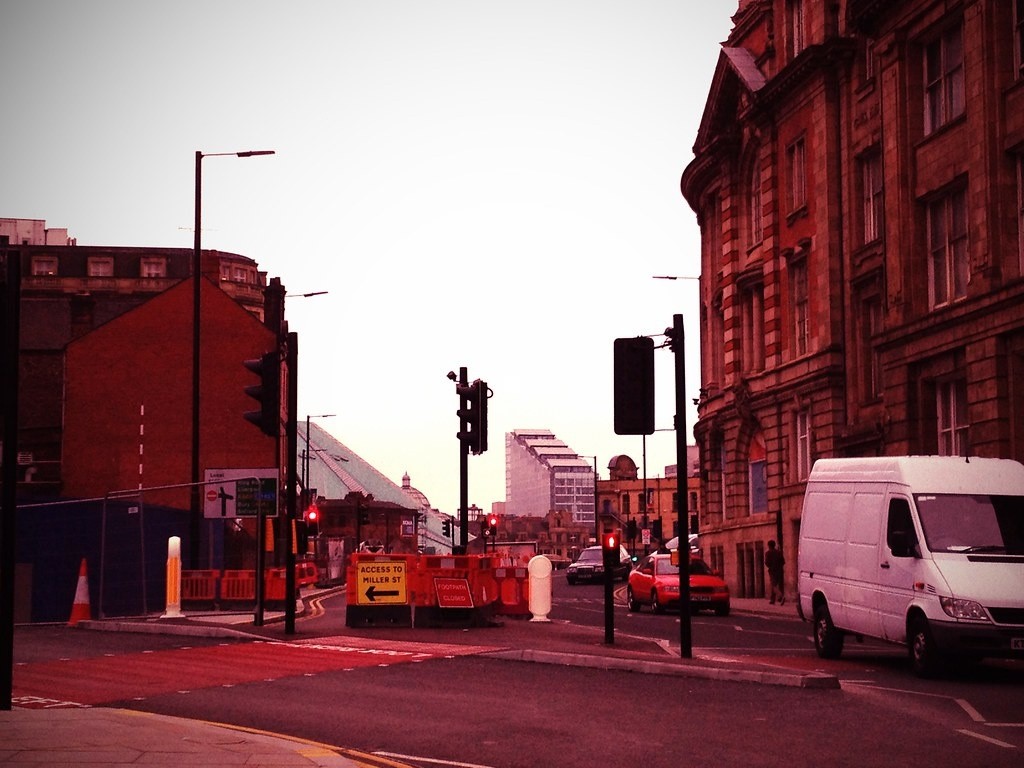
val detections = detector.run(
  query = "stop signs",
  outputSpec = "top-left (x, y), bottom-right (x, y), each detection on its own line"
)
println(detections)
top-left (206, 490), bottom-right (218, 501)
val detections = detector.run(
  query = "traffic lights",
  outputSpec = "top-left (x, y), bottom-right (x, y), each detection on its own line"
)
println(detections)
top-left (357, 503), bottom-right (370, 526)
top-left (602, 532), bottom-right (621, 568)
top-left (243, 351), bottom-right (278, 437)
top-left (455, 379), bottom-right (488, 455)
top-left (488, 517), bottom-right (496, 535)
top-left (442, 519), bottom-right (450, 538)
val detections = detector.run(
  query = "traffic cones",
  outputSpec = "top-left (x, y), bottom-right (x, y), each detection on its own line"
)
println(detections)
top-left (64, 556), bottom-right (93, 627)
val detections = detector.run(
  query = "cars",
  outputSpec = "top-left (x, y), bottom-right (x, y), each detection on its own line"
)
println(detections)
top-left (647, 533), bottom-right (699, 558)
top-left (566, 545), bottom-right (633, 586)
top-left (626, 552), bottom-right (729, 617)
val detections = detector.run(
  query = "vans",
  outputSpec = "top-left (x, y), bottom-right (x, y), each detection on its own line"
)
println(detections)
top-left (796, 453), bottom-right (1024, 679)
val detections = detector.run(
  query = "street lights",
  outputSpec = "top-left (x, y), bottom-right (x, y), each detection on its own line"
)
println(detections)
top-left (184, 149), bottom-right (276, 610)
top-left (300, 449), bottom-right (327, 554)
top-left (304, 413), bottom-right (337, 552)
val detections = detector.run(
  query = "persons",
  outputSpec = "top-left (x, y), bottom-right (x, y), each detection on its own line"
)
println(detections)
top-left (765, 540), bottom-right (786, 607)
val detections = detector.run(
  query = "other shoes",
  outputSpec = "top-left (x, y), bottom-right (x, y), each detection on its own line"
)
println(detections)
top-left (770, 602), bottom-right (774, 605)
top-left (780, 596), bottom-right (785, 605)
